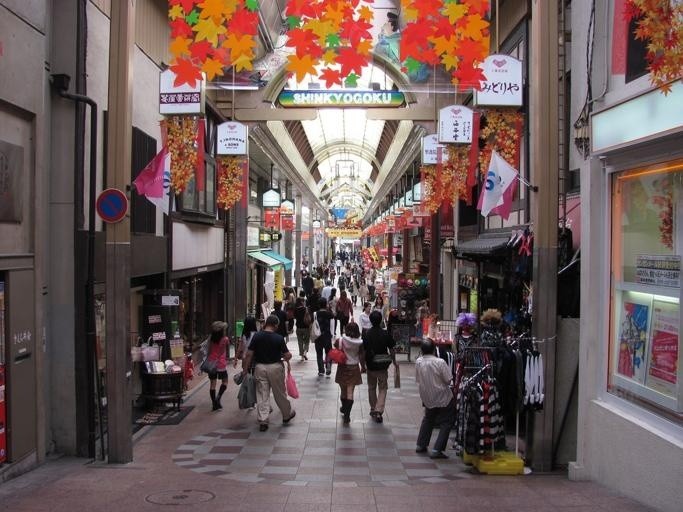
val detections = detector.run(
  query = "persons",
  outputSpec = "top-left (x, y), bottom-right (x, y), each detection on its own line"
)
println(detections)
top-left (199, 320), bottom-right (230, 411)
top-left (414, 337), bottom-right (457, 460)
top-left (233, 250), bottom-right (399, 431)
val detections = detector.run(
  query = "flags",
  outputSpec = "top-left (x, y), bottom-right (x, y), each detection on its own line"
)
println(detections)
top-left (133, 145), bottom-right (177, 216)
top-left (476, 150), bottom-right (520, 221)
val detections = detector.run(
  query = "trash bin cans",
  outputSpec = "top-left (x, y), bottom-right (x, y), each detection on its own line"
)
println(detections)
top-left (368, 286), bottom-right (375, 301)
top-left (313, 280), bottom-right (325, 292)
top-left (176, 351), bottom-right (193, 391)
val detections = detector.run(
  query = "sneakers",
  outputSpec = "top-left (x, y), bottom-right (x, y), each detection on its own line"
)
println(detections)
top-left (370, 411), bottom-right (376, 417)
top-left (283, 411), bottom-right (295, 423)
top-left (416, 445), bottom-right (428, 453)
top-left (259, 424), bottom-right (268, 431)
top-left (430, 450), bottom-right (449, 459)
top-left (375, 412), bottom-right (382, 423)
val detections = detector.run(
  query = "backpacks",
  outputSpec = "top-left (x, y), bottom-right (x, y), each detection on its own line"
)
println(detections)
top-left (303, 307), bottom-right (312, 326)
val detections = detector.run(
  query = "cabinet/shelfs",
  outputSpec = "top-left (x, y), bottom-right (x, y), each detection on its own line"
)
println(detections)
top-left (141, 369), bottom-right (185, 412)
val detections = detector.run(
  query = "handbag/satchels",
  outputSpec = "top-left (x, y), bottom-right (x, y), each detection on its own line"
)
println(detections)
top-left (310, 312), bottom-right (322, 342)
top-left (131, 336), bottom-right (144, 361)
top-left (286, 361), bottom-right (299, 399)
top-left (141, 336), bottom-right (163, 361)
top-left (372, 354), bottom-right (392, 369)
top-left (200, 359), bottom-right (217, 374)
top-left (336, 311), bottom-right (344, 320)
top-left (328, 337), bottom-right (346, 365)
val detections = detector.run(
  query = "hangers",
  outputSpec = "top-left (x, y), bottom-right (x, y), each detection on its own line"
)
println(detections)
top-left (458, 329), bottom-right (545, 403)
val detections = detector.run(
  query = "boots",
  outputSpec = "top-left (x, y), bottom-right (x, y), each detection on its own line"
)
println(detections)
top-left (344, 399), bottom-right (354, 423)
top-left (340, 398), bottom-right (347, 413)
top-left (216, 384), bottom-right (227, 408)
top-left (210, 389), bottom-right (222, 410)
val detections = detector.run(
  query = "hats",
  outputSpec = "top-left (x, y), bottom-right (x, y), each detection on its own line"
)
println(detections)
top-left (212, 321), bottom-right (228, 331)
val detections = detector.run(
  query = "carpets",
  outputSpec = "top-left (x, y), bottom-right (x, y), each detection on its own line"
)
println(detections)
top-left (134, 405), bottom-right (195, 426)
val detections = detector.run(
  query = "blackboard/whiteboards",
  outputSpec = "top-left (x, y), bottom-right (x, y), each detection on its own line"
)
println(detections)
top-left (388, 324), bottom-right (412, 354)
